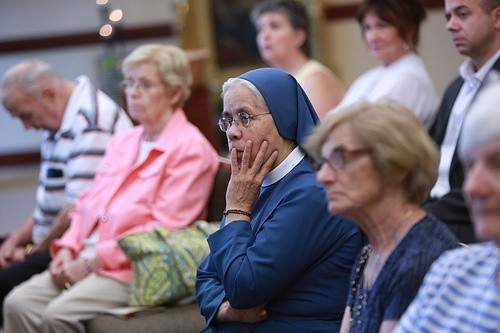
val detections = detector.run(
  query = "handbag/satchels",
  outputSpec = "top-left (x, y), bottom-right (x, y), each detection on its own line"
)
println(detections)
top-left (117, 219), bottom-right (221, 307)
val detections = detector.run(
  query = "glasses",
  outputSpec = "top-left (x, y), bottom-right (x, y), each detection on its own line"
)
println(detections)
top-left (311, 144), bottom-right (375, 170)
top-left (217, 112), bottom-right (271, 133)
top-left (119, 79), bottom-right (168, 94)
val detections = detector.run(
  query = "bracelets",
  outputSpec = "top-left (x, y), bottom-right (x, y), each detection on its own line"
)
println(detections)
top-left (82, 248), bottom-right (104, 275)
top-left (25, 244), bottom-right (32, 263)
top-left (223, 209), bottom-right (252, 217)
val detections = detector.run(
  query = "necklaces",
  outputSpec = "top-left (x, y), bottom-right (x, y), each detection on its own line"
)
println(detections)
top-left (348, 247), bottom-right (373, 327)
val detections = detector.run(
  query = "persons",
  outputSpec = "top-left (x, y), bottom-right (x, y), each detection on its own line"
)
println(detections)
top-left (392, 115), bottom-right (500, 333)
top-left (249, 0), bottom-right (346, 123)
top-left (324, 0), bottom-right (440, 133)
top-left (0, 61), bottom-right (135, 329)
top-left (300, 98), bottom-right (466, 333)
top-left (2, 45), bottom-right (220, 333)
top-left (194, 67), bottom-right (364, 333)
top-left (428, 0), bottom-right (500, 243)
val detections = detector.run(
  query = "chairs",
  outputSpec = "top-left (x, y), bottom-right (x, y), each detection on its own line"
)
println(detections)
top-left (85, 169), bottom-right (231, 332)
